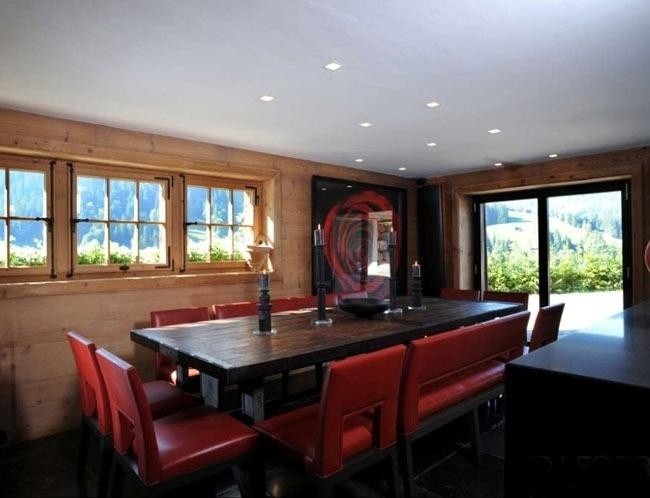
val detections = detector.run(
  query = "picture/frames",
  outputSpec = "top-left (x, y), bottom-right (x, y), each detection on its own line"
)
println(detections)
top-left (311, 174), bottom-right (408, 296)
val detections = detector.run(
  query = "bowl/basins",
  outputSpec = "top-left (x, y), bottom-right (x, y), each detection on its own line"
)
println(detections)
top-left (337, 298), bottom-right (392, 317)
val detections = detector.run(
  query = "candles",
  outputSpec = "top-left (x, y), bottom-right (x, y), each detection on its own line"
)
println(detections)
top-left (411, 260), bottom-right (421, 278)
top-left (314, 224), bottom-right (324, 246)
top-left (388, 226), bottom-right (396, 244)
top-left (259, 268), bottom-right (269, 289)
